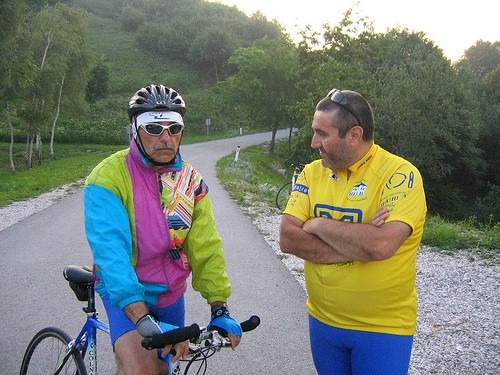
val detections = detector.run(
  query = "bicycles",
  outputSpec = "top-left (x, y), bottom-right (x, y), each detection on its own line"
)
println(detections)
top-left (18, 264), bottom-right (261, 375)
top-left (275, 163), bottom-right (306, 211)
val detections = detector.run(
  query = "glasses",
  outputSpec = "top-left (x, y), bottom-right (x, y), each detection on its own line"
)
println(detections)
top-left (139, 124), bottom-right (184, 136)
top-left (328, 88), bottom-right (363, 127)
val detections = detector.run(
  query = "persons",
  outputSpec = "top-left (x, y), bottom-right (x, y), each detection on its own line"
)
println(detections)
top-left (83, 83), bottom-right (243, 375)
top-left (279, 89), bottom-right (426, 375)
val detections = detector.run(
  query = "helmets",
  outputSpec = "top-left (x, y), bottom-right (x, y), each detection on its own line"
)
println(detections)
top-left (128, 84), bottom-right (187, 120)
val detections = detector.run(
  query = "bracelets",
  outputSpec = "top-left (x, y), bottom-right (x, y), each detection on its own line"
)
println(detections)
top-left (211, 302), bottom-right (226, 312)
